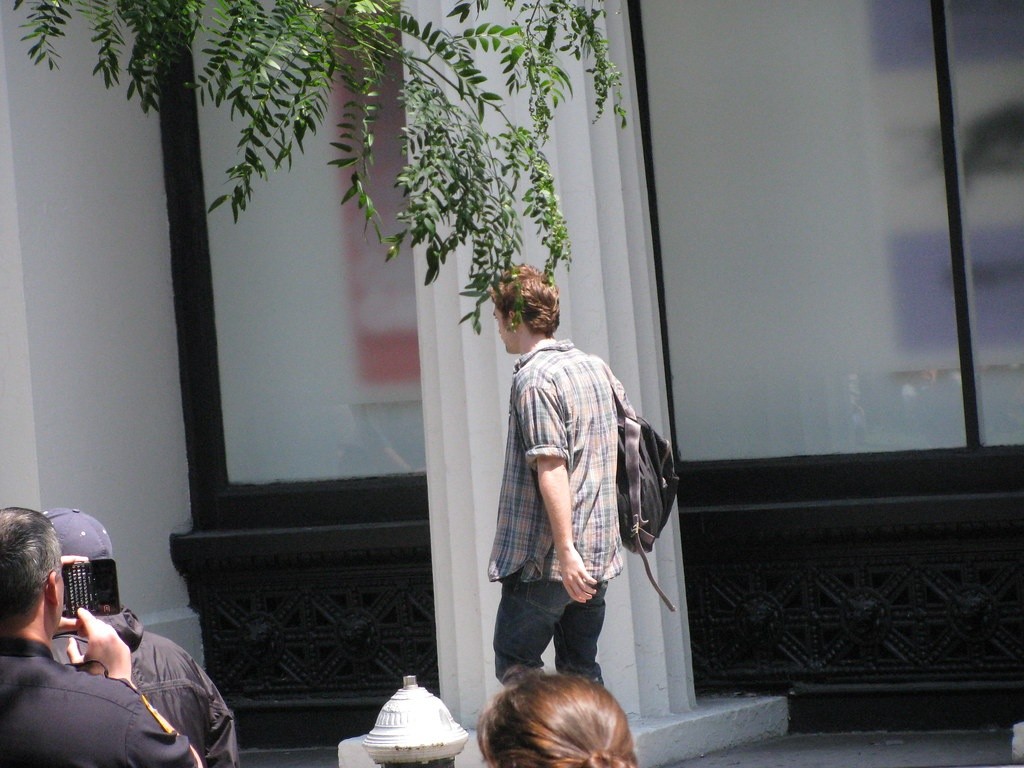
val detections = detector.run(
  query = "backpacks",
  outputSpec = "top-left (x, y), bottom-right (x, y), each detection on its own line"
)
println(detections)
top-left (590, 354), bottom-right (679, 554)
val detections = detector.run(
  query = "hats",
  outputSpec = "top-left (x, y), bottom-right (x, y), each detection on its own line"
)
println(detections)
top-left (40, 508), bottom-right (112, 561)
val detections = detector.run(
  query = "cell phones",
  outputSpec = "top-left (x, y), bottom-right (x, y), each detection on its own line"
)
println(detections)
top-left (61, 562), bottom-right (100, 618)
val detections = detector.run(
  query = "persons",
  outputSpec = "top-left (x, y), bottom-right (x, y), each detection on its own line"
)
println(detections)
top-left (0, 507), bottom-right (239, 768)
top-left (486, 267), bottom-right (637, 688)
top-left (477, 665), bottom-right (638, 768)
